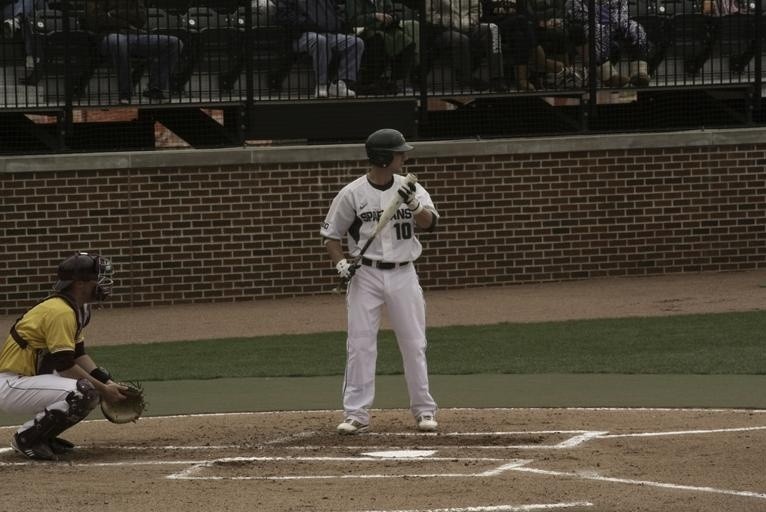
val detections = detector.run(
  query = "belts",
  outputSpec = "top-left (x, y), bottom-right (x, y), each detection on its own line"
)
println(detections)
top-left (360, 258), bottom-right (410, 271)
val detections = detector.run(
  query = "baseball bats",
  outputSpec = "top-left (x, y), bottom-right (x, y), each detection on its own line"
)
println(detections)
top-left (332, 173), bottom-right (418, 295)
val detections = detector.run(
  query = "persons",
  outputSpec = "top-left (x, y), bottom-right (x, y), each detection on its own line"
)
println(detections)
top-left (1, 1), bottom-right (766, 102)
top-left (0, 258), bottom-right (129, 462)
top-left (319, 129), bottom-right (439, 435)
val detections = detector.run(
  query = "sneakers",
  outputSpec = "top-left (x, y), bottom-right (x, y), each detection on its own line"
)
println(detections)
top-left (415, 411), bottom-right (439, 431)
top-left (9, 431), bottom-right (61, 465)
top-left (336, 419), bottom-right (369, 435)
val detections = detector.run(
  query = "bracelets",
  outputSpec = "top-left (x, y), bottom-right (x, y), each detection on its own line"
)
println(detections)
top-left (408, 200), bottom-right (425, 215)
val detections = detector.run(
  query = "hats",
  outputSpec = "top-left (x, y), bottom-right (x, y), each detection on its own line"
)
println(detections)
top-left (363, 129), bottom-right (416, 167)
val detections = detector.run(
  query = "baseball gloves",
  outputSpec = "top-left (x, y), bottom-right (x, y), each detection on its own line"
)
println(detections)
top-left (101, 383), bottom-right (145, 424)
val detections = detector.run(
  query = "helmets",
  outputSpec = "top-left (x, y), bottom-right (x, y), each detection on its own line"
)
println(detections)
top-left (49, 251), bottom-right (112, 297)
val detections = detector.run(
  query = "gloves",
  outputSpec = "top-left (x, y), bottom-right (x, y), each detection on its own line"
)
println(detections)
top-left (334, 260), bottom-right (363, 282)
top-left (396, 183), bottom-right (425, 217)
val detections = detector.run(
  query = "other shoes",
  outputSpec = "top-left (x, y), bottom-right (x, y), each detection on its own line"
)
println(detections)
top-left (605, 75), bottom-right (630, 87)
top-left (631, 73), bottom-right (651, 86)
top-left (71, 75), bottom-right (87, 98)
top-left (52, 63), bottom-right (68, 75)
top-left (456, 67), bottom-right (588, 91)
top-left (269, 76), bottom-right (283, 91)
top-left (314, 69), bottom-right (421, 99)
top-left (222, 73), bottom-right (236, 88)
top-left (118, 86), bottom-right (189, 100)
